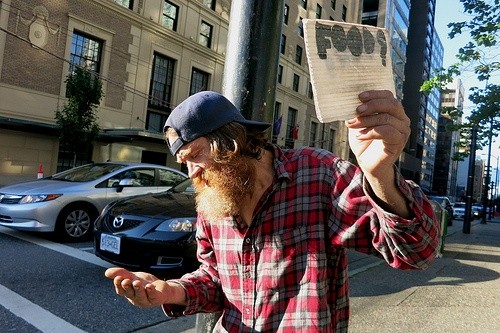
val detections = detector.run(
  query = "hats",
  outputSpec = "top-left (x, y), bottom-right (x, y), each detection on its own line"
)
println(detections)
top-left (163, 90), bottom-right (273, 155)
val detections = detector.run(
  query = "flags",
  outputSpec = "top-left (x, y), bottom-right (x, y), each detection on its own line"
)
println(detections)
top-left (273, 116), bottom-right (282, 136)
top-left (292, 121), bottom-right (299, 140)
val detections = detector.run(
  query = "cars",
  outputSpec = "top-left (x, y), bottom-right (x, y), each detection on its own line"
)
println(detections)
top-left (452, 202), bottom-right (466, 220)
top-left (469, 205), bottom-right (484, 220)
top-left (0, 162), bottom-right (190, 240)
top-left (91, 178), bottom-right (205, 272)
top-left (430, 196), bottom-right (453, 226)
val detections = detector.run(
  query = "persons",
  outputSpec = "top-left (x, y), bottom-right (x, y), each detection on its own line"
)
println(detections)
top-left (104, 90), bottom-right (439, 333)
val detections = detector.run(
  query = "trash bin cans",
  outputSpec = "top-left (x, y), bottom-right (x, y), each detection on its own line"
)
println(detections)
top-left (428, 197), bottom-right (454, 258)
